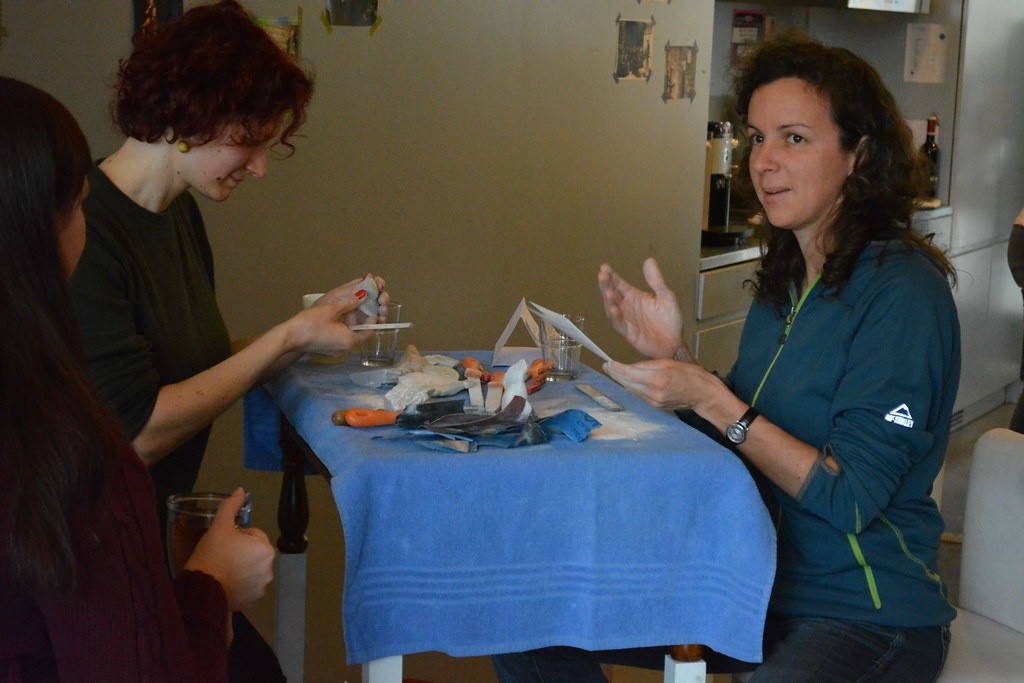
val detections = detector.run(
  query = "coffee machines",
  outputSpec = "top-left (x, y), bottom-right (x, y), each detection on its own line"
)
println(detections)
top-left (701, 116), bottom-right (755, 245)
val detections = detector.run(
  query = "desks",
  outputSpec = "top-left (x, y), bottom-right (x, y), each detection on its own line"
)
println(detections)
top-left (243, 343), bottom-right (776, 683)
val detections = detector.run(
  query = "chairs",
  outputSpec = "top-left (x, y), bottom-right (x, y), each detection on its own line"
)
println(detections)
top-left (930, 427), bottom-right (1023, 683)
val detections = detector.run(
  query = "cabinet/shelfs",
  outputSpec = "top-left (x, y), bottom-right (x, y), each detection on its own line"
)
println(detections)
top-left (695, 205), bottom-right (952, 379)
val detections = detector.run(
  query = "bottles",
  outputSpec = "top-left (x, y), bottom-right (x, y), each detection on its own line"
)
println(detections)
top-left (910, 112), bottom-right (943, 210)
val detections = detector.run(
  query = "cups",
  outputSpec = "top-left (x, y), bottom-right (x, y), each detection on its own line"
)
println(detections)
top-left (160, 486), bottom-right (254, 589)
top-left (539, 309), bottom-right (585, 381)
top-left (360, 302), bottom-right (403, 366)
top-left (302, 291), bottom-right (350, 364)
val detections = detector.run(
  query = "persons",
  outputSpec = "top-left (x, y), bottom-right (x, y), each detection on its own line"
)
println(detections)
top-left (0, 75), bottom-right (278, 682)
top-left (1006, 205), bottom-right (1024, 435)
top-left (598, 27), bottom-right (961, 683)
top-left (57, 0), bottom-right (394, 683)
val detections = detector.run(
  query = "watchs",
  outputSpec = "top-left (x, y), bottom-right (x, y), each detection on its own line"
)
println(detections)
top-left (721, 406), bottom-right (761, 450)
